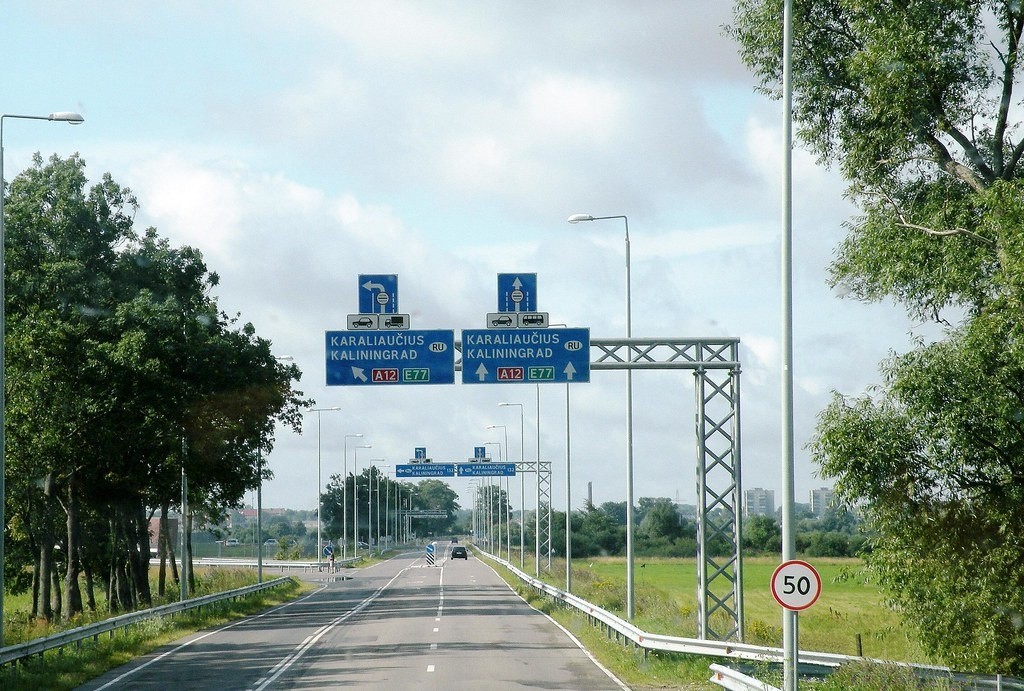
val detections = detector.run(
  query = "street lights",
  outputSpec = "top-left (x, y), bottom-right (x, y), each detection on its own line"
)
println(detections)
top-left (497, 402), bottom-right (526, 567)
top-left (569, 211), bottom-right (640, 619)
top-left (481, 440), bottom-right (502, 559)
top-left (178, 356), bottom-right (293, 602)
top-left (368, 457), bottom-right (386, 555)
top-left (303, 405), bottom-right (343, 560)
top-left (354, 444), bottom-right (371, 557)
top-left (486, 424), bottom-right (512, 563)
top-left (342, 434), bottom-right (364, 559)
top-left (0, 108), bottom-right (86, 645)
top-left (377, 464), bottom-right (390, 552)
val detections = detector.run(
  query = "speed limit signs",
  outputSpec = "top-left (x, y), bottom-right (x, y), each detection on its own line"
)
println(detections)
top-left (771, 561), bottom-right (820, 615)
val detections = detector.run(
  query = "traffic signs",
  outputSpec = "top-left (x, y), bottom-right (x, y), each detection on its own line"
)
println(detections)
top-left (460, 328), bottom-right (590, 386)
top-left (324, 328), bottom-right (455, 386)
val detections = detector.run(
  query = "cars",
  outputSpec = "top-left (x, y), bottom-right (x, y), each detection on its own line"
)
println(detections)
top-left (452, 536), bottom-right (458, 544)
top-left (263, 538), bottom-right (279, 547)
top-left (225, 539), bottom-right (239, 548)
top-left (357, 541), bottom-right (370, 551)
top-left (451, 548), bottom-right (469, 561)
